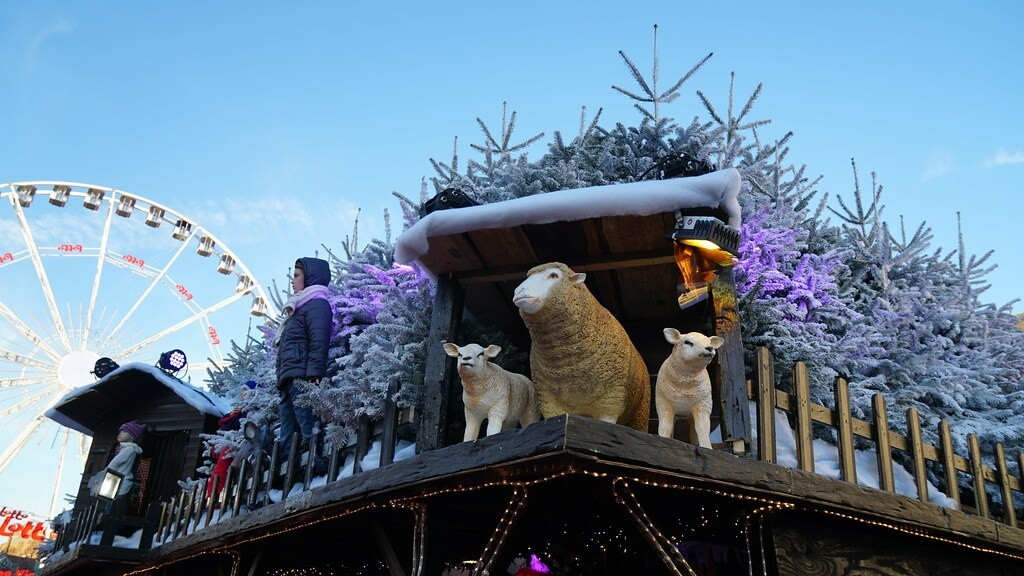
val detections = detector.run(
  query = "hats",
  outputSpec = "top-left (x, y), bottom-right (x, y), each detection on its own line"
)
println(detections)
top-left (119, 420), bottom-right (146, 442)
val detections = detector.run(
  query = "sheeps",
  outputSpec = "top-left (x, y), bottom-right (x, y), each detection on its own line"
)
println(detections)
top-left (440, 262), bottom-right (729, 442)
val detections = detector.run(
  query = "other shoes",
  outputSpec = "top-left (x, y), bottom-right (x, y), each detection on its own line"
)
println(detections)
top-left (247, 498), bottom-right (275, 510)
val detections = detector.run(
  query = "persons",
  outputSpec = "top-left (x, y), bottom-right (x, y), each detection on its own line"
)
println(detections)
top-left (205, 380), bottom-right (263, 507)
top-left (87, 419), bottom-right (147, 530)
top-left (252, 257), bottom-right (332, 462)
